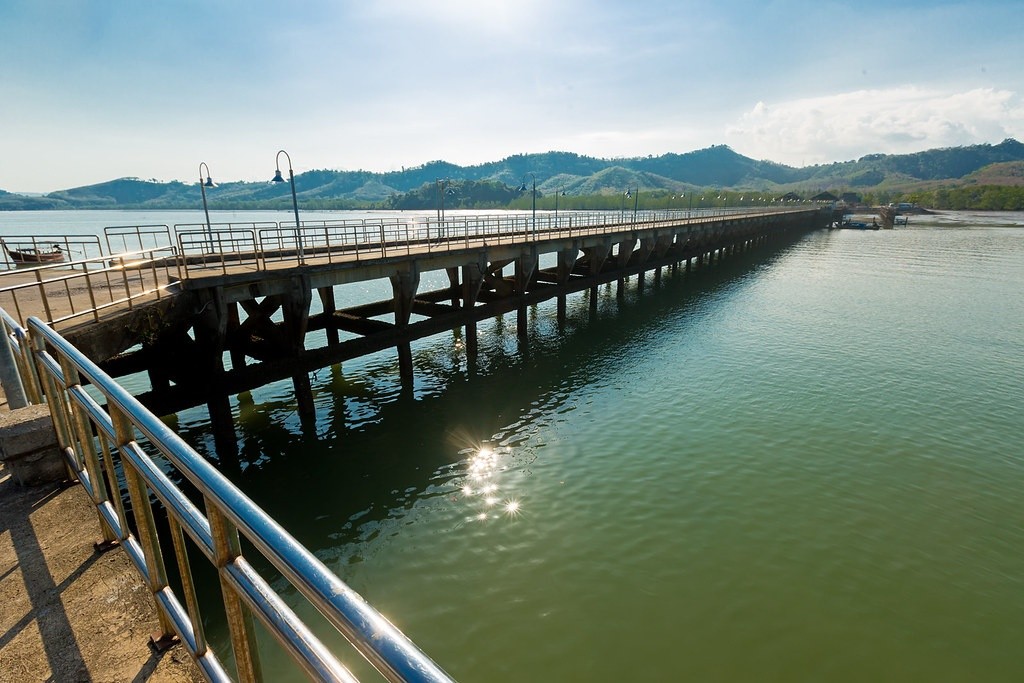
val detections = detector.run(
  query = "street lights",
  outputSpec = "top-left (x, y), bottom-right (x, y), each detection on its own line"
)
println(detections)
top-left (199, 162), bottom-right (218, 254)
top-left (619, 182), bottom-right (839, 226)
top-left (441, 176), bottom-right (454, 239)
top-left (270, 150), bottom-right (309, 268)
top-left (554, 184), bottom-right (566, 228)
top-left (518, 171), bottom-right (536, 241)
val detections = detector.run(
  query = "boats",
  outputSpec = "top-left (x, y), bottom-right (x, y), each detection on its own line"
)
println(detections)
top-left (826, 213), bottom-right (881, 231)
top-left (0, 238), bottom-right (81, 264)
